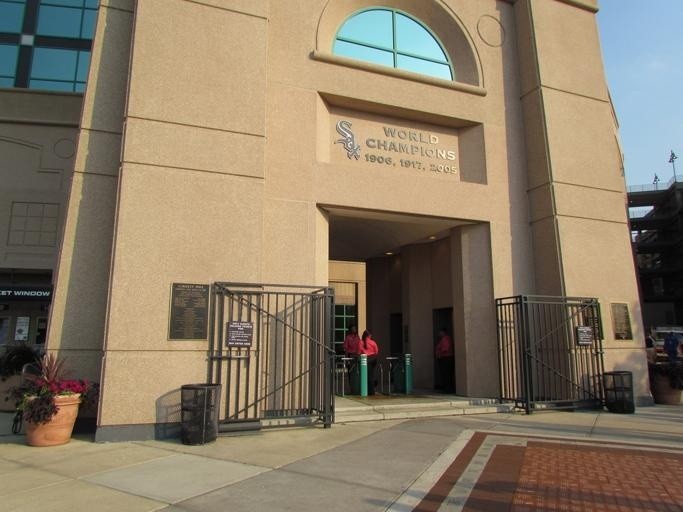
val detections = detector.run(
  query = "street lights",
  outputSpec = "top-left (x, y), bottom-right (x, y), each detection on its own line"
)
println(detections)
top-left (667, 149), bottom-right (678, 184)
top-left (652, 173), bottom-right (660, 190)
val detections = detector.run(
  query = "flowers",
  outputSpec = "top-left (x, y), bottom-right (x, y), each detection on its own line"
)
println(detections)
top-left (3, 352), bottom-right (99, 435)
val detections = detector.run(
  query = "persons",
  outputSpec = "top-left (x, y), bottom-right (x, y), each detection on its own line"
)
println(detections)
top-left (646, 333), bottom-right (655, 348)
top-left (435, 327), bottom-right (452, 394)
top-left (356, 330), bottom-right (379, 395)
top-left (343, 324), bottom-right (360, 394)
top-left (663, 332), bottom-right (679, 368)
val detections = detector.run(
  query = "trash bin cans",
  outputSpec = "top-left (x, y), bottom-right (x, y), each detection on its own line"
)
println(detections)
top-left (603, 371), bottom-right (635, 414)
top-left (181, 383), bottom-right (222, 445)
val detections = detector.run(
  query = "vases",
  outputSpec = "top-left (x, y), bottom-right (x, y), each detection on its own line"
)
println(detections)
top-left (24, 394), bottom-right (81, 446)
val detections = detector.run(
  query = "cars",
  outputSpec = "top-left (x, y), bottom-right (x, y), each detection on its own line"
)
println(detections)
top-left (645, 325), bottom-right (683, 368)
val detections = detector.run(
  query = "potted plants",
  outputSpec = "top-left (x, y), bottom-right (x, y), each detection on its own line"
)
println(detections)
top-left (648, 355), bottom-right (683, 405)
